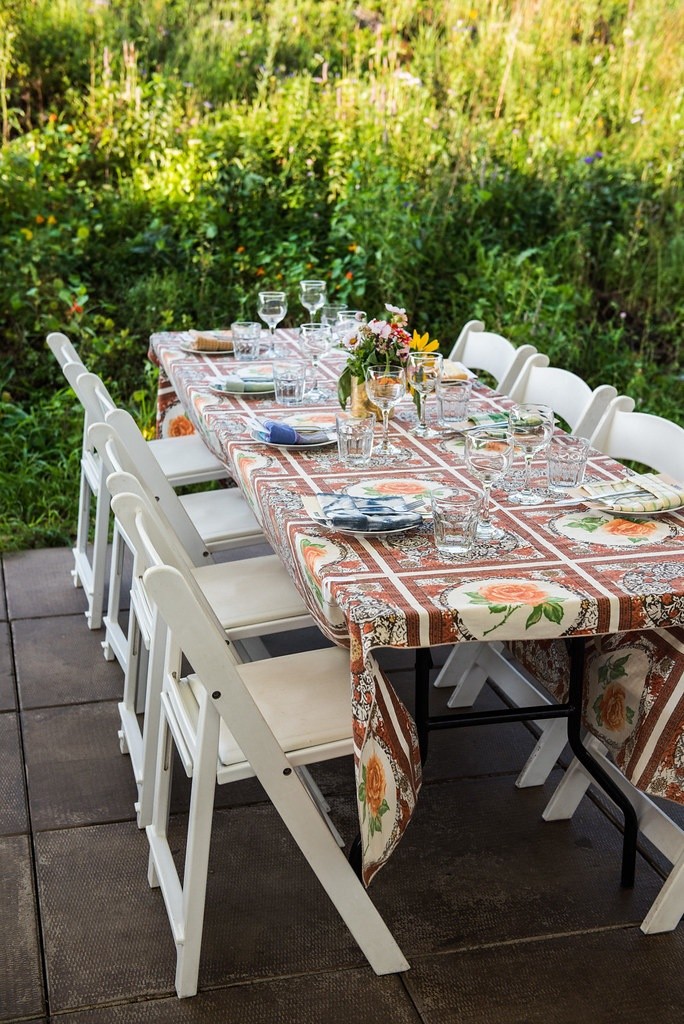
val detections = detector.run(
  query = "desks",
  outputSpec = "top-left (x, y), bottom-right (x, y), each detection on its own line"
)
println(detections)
top-left (148, 331), bottom-right (684, 896)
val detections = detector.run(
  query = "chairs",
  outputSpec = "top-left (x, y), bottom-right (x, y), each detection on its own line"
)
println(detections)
top-left (48, 320), bottom-right (684, 999)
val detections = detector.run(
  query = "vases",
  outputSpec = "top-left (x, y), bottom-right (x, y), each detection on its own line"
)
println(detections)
top-left (351, 374), bottom-right (395, 420)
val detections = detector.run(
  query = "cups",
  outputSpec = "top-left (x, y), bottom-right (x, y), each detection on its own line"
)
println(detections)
top-left (546, 434), bottom-right (591, 493)
top-left (320, 302), bottom-right (367, 352)
top-left (430, 486), bottom-right (483, 555)
top-left (230, 321), bottom-right (262, 362)
top-left (435, 378), bottom-right (473, 428)
top-left (335, 410), bottom-right (376, 470)
top-left (273, 359), bottom-right (306, 407)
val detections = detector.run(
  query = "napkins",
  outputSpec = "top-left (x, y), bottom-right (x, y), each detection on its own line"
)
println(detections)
top-left (254, 416), bottom-right (329, 443)
top-left (186, 329), bottom-right (233, 350)
top-left (211, 375), bottom-right (275, 392)
top-left (316, 493), bottom-right (423, 530)
top-left (579, 472), bottom-right (684, 512)
top-left (442, 359), bottom-right (468, 379)
top-left (468, 412), bottom-right (509, 429)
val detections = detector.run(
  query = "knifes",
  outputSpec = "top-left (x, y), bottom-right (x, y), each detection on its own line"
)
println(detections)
top-left (554, 488), bottom-right (645, 506)
top-left (438, 421), bottom-right (509, 434)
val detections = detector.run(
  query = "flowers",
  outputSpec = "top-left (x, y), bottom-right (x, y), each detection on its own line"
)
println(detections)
top-left (338, 303), bottom-right (439, 411)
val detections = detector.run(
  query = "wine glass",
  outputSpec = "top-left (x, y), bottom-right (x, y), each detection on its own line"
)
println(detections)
top-left (506, 403), bottom-right (554, 506)
top-left (365, 364), bottom-right (406, 455)
top-left (298, 323), bottom-right (333, 405)
top-left (463, 429), bottom-right (514, 540)
top-left (256, 289), bottom-right (288, 359)
top-left (405, 352), bottom-right (444, 438)
top-left (299, 281), bottom-right (327, 341)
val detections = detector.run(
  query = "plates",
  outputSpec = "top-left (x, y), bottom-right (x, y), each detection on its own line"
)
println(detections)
top-left (178, 341), bottom-right (239, 355)
top-left (314, 519), bottom-right (420, 539)
top-left (250, 430), bottom-right (338, 450)
top-left (602, 504), bottom-right (684, 518)
top-left (207, 384), bottom-right (284, 397)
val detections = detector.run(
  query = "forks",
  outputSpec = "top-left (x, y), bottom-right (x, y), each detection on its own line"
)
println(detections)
top-left (597, 493), bottom-right (654, 504)
